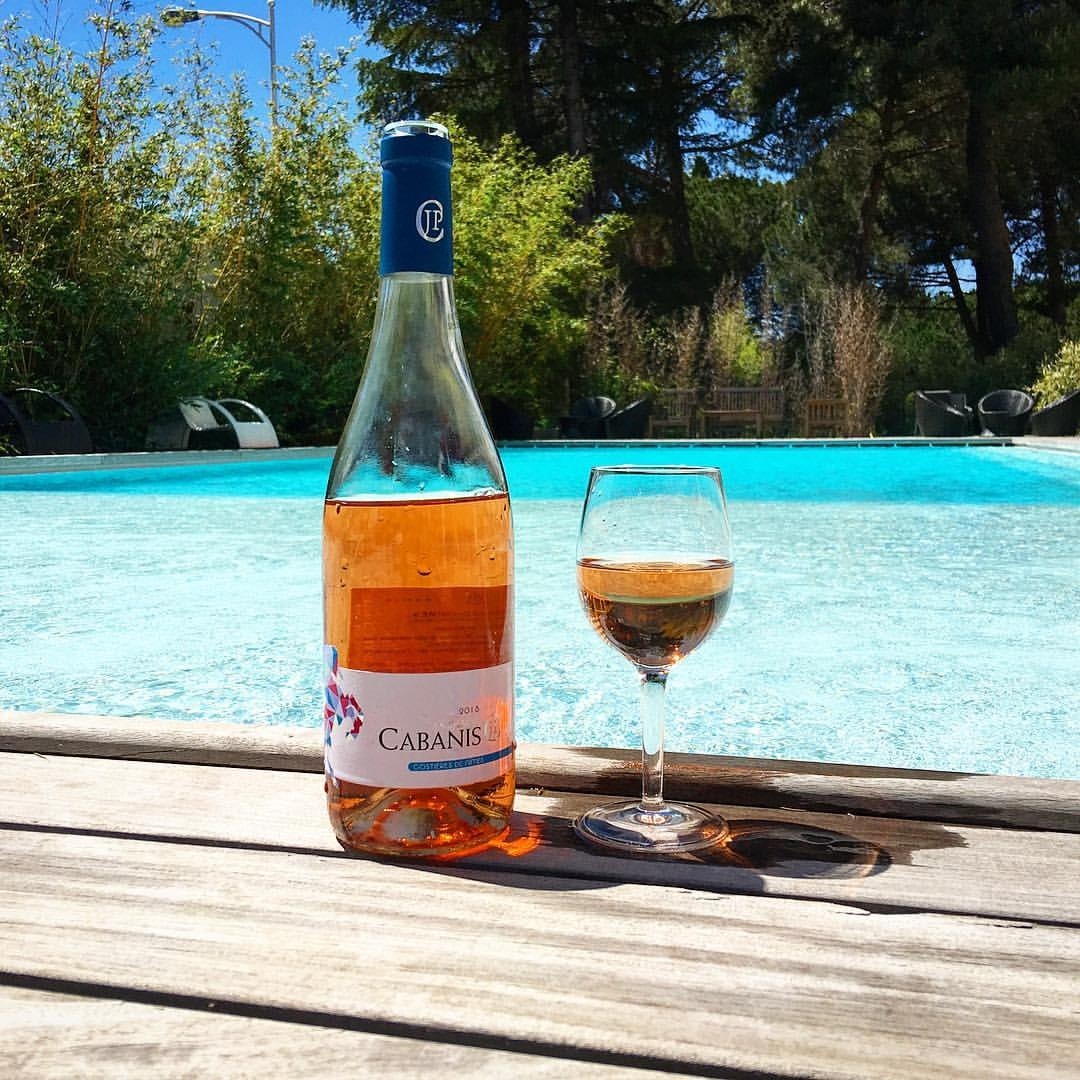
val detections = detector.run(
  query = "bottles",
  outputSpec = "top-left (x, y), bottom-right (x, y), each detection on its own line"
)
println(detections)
top-left (321, 119), bottom-right (514, 861)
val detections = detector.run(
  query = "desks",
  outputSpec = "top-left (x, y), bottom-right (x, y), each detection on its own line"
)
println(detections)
top-left (697, 408), bottom-right (764, 439)
top-left (0, 718), bottom-right (1077, 1077)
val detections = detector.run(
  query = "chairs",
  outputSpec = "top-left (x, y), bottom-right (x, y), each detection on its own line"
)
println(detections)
top-left (914, 388), bottom-right (1080, 437)
top-left (483, 393), bottom-right (653, 442)
top-left (0, 387), bottom-right (280, 458)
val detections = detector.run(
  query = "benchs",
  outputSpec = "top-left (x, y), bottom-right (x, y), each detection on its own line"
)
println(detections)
top-left (643, 385), bottom-right (855, 439)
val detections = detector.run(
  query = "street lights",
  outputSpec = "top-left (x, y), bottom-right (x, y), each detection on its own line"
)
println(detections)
top-left (158, 0), bottom-right (279, 126)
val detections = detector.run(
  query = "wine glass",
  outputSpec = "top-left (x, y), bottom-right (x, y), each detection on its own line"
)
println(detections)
top-left (574, 464), bottom-right (736, 856)
